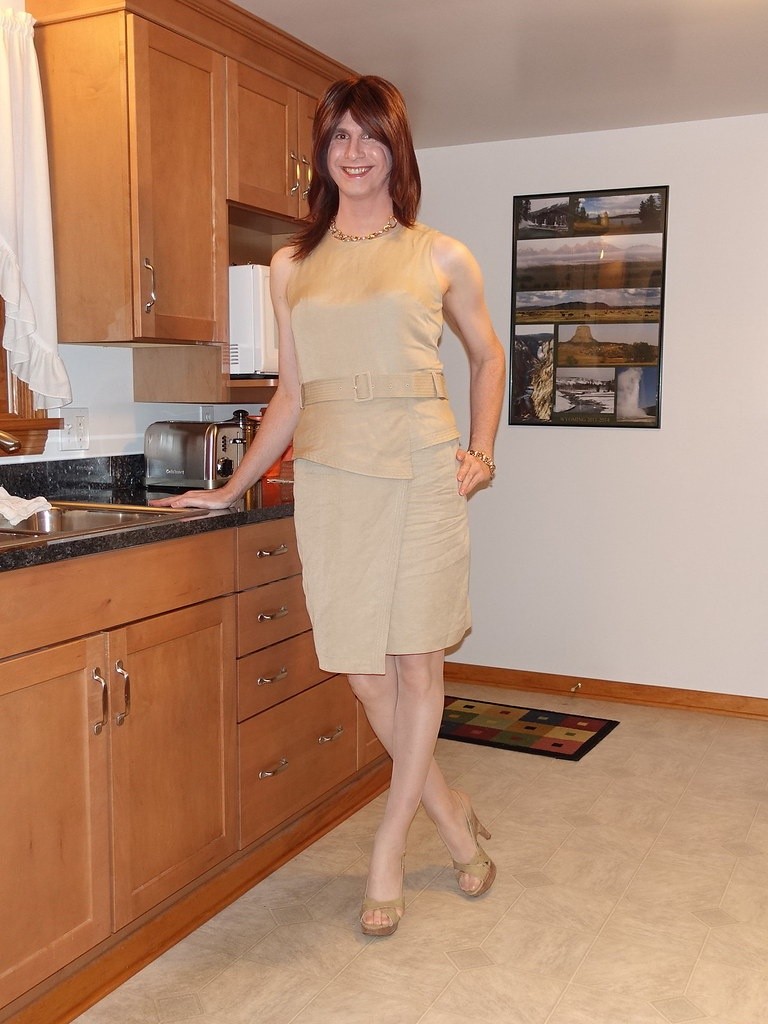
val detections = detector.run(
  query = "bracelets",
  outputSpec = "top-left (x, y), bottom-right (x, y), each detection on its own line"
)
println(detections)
top-left (468, 449), bottom-right (497, 481)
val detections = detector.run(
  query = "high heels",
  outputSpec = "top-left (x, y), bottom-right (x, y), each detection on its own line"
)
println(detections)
top-left (360, 849), bottom-right (405, 936)
top-left (436, 790), bottom-right (495, 898)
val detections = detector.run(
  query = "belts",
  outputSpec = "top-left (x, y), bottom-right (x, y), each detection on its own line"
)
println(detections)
top-left (301, 372), bottom-right (449, 405)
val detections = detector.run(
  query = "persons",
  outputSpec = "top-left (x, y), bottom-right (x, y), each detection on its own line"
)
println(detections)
top-left (145, 74), bottom-right (505, 938)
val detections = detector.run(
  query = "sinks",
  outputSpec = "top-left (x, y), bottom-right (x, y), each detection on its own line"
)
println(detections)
top-left (1, 526), bottom-right (56, 553)
top-left (0, 500), bottom-right (211, 533)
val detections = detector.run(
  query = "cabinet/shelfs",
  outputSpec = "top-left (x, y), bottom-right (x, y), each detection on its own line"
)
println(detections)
top-left (0, 511), bottom-right (393, 1023)
top-left (24, 0), bottom-right (369, 344)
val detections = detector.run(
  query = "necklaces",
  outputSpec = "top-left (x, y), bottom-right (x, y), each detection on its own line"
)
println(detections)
top-left (327, 214), bottom-right (398, 242)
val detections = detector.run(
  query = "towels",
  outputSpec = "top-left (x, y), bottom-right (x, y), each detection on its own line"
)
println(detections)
top-left (0, 486), bottom-right (52, 526)
